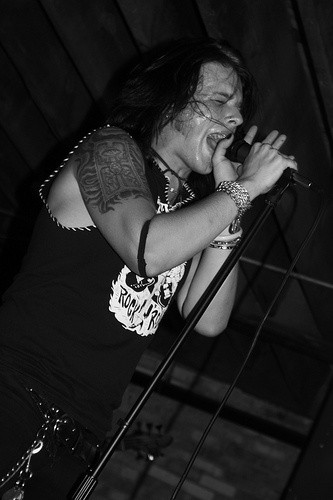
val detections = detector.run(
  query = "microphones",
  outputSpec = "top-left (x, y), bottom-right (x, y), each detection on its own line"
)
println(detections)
top-left (224, 137), bottom-right (311, 189)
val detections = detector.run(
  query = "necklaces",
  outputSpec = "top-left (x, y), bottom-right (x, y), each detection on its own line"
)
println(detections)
top-left (150, 146), bottom-right (187, 208)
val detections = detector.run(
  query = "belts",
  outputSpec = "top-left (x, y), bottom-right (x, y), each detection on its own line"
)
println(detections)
top-left (18, 386), bottom-right (97, 462)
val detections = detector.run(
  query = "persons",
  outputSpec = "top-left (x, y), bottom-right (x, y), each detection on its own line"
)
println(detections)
top-left (0, 39), bottom-right (300, 500)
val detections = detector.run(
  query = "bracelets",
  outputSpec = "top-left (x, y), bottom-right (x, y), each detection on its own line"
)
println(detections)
top-left (208, 227), bottom-right (243, 250)
top-left (216, 181), bottom-right (252, 235)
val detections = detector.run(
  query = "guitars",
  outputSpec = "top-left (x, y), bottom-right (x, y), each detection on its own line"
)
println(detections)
top-left (104, 421), bottom-right (174, 459)
